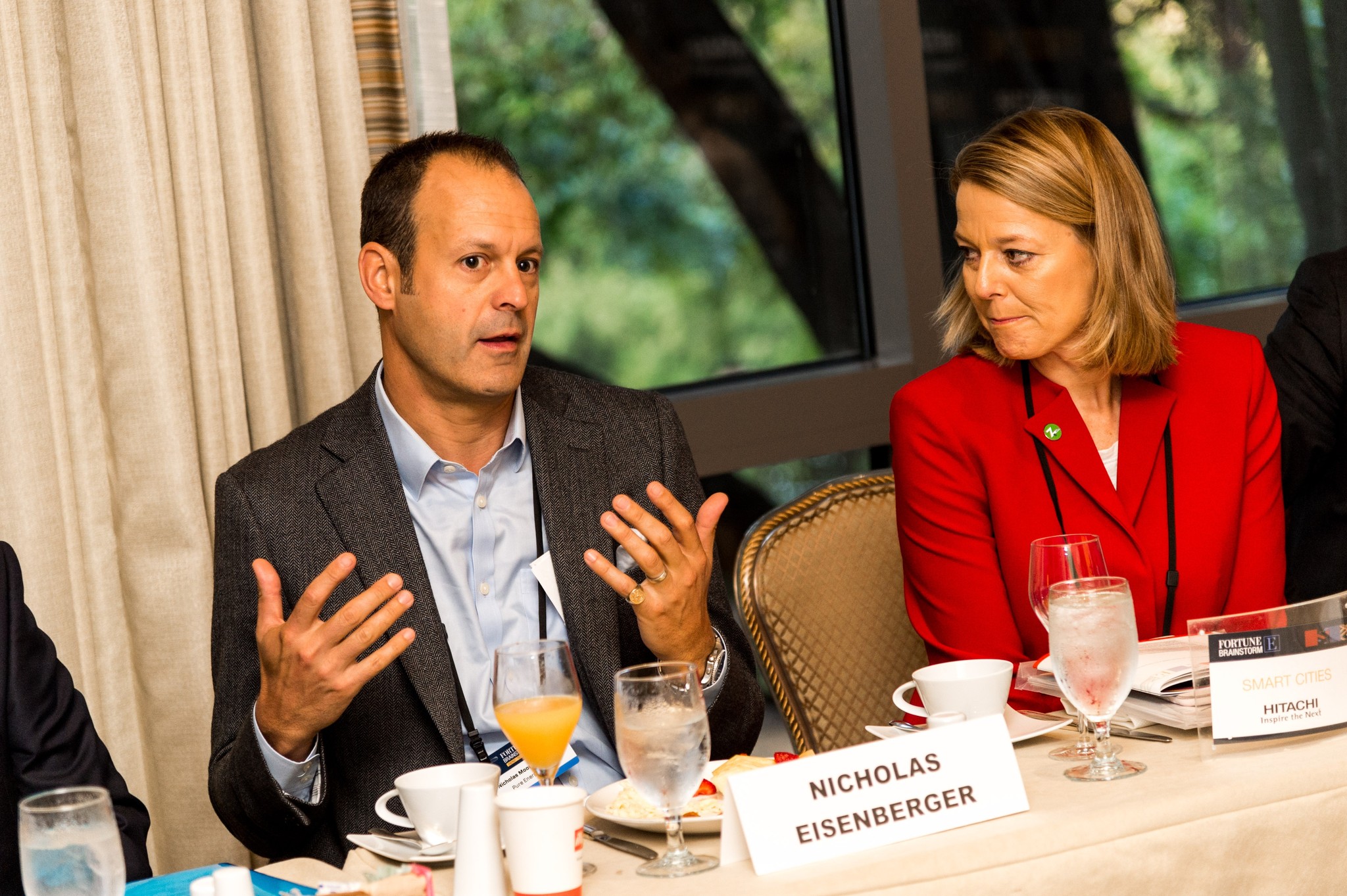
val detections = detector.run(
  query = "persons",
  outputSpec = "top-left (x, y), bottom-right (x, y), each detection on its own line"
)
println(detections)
top-left (209, 128), bottom-right (766, 871)
top-left (887, 105), bottom-right (1291, 730)
top-left (0, 539), bottom-right (156, 896)
top-left (1257, 241), bottom-right (1346, 649)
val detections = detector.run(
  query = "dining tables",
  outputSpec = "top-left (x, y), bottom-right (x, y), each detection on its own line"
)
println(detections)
top-left (252, 695), bottom-right (1347, 896)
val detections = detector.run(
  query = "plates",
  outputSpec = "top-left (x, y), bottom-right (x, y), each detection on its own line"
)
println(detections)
top-left (346, 829), bottom-right (504, 861)
top-left (865, 700), bottom-right (1073, 744)
top-left (584, 757), bottom-right (779, 834)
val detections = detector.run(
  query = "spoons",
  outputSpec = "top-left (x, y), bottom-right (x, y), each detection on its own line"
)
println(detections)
top-left (368, 827), bottom-right (455, 857)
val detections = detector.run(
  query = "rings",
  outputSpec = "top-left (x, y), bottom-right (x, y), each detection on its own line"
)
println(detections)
top-left (645, 564), bottom-right (670, 583)
top-left (623, 583), bottom-right (647, 605)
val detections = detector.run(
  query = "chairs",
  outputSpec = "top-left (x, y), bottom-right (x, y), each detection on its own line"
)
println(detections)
top-left (732, 465), bottom-right (929, 759)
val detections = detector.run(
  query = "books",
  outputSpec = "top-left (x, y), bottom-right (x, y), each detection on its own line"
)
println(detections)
top-left (1032, 632), bottom-right (1233, 698)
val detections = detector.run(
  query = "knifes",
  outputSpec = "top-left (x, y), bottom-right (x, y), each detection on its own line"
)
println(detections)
top-left (1017, 709), bottom-right (1174, 742)
top-left (583, 824), bottom-right (659, 860)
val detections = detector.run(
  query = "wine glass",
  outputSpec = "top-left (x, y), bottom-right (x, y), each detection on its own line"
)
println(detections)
top-left (1028, 533), bottom-right (1126, 761)
top-left (1048, 576), bottom-right (1147, 781)
top-left (613, 660), bottom-right (719, 876)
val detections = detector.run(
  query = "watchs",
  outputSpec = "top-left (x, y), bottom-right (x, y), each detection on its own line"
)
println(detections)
top-left (657, 630), bottom-right (727, 694)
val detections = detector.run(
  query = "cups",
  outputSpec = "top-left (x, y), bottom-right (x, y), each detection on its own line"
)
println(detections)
top-left (492, 641), bottom-right (583, 785)
top-left (17, 786), bottom-right (127, 896)
top-left (892, 659), bottom-right (1014, 721)
top-left (495, 785), bottom-right (589, 896)
top-left (375, 762), bottom-right (501, 846)
